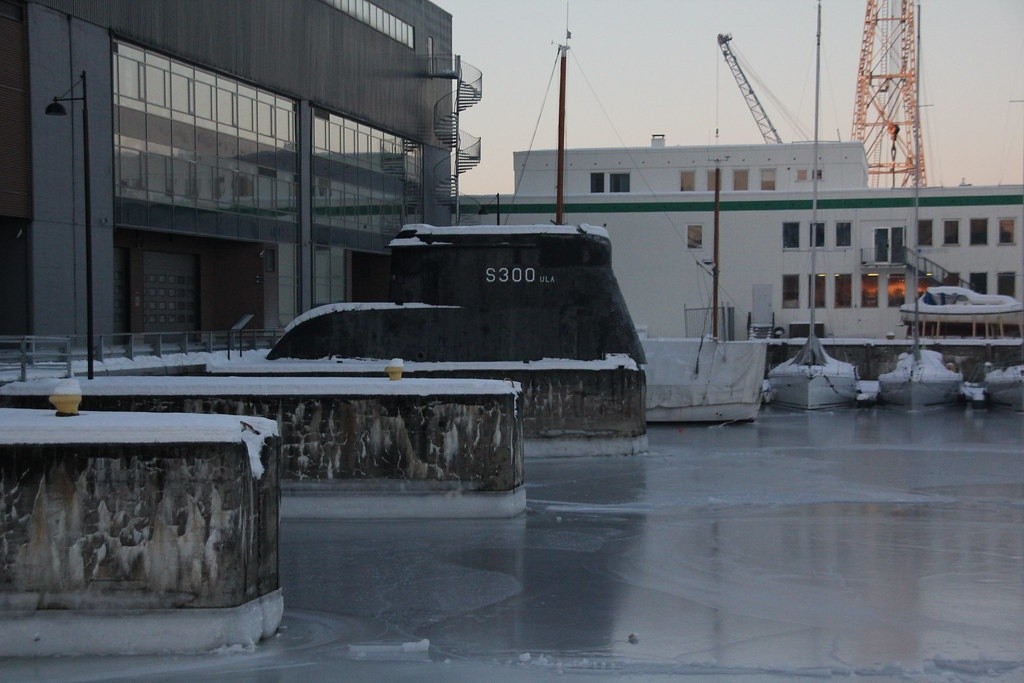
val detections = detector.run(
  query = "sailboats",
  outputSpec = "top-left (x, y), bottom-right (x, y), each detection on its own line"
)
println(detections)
top-left (878, 3), bottom-right (964, 409)
top-left (766, 2), bottom-right (863, 409)
top-left (505, 2), bottom-right (776, 423)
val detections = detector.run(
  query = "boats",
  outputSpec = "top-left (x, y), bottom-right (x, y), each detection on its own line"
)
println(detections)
top-left (898, 285), bottom-right (1023, 326)
